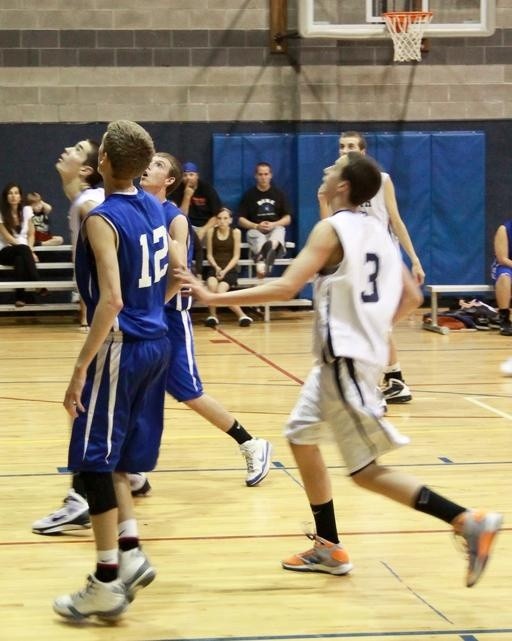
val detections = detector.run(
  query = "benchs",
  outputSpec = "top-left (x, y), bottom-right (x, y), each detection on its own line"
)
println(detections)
top-left (424, 285), bottom-right (497, 336)
top-left (0, 242), bottom-right (311, 323)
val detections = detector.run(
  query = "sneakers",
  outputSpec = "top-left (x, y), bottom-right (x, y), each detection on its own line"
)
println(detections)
top-left (14, 300), bottom-right (27, 308)
top-left (206, 316), bottom-right (219, 326)
top-left (239, 436), bottom-right (272, 486)
top-left (123, 470), bottom-right (153, 498)
top-left (501, 357), bottom-right (512, 378)
top-left (238, 316), bottom-right (253, 327)
top-left (36, 288), bottom-right (51, 299)
top-left (255, 240), bottom-right (272, 263)
top-left (498, 309), bottom-right (512, 335)
top-left (456, 507), bottom-right (502, 587)
top-left (282, 535), bottom-right (354, 575)
top-left (383, 377), bottom-right (411, 401)
top-left (32, 489), bottom-right (95, 535)
top-left (54, 542), bottom-right (156, 623)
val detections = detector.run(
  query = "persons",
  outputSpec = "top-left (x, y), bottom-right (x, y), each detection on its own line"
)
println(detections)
top-left (237, 162), bottom-right (293, 277)
top-left (176, 161), bottom-right (222, 245)
top-left (173, 152), bottom-right (505, 587)
top-left (128, 151), bottom-right (273, 498)
top-left (317, 129), bottom-right (425, 403)
top-left (26, 191), bottom-right (64, 246)
top-left (52, 118), bottom-right (189, 624)
top-left (31, 137), bottom-right (108, 535)
top-left (489, 219), bottom-right (512, 336)
top-left (206, 206), bottom-right (253, 327)
top-left (0, 182), bottom-right (48, 306)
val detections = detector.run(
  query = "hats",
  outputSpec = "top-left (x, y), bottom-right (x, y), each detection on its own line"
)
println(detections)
top-left (182, 162), bottom-right (198, 173)
